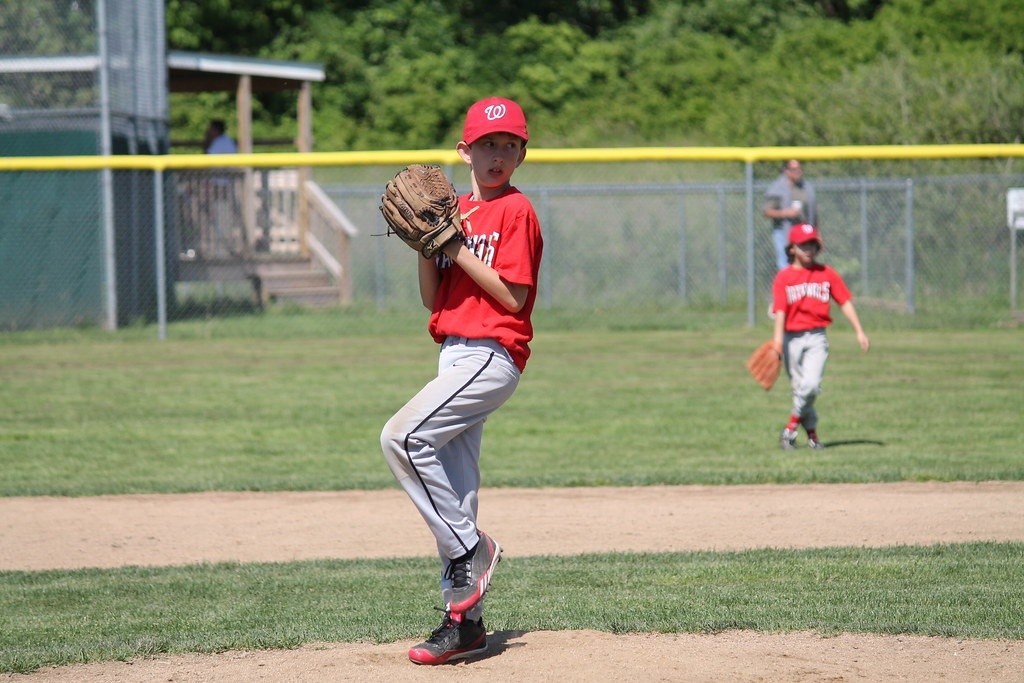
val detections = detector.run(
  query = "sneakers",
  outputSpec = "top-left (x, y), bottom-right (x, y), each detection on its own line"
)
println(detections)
top-left (450, 528), bottom-right (503, 613)
top-left (409, 614), bottom-right (489, 666)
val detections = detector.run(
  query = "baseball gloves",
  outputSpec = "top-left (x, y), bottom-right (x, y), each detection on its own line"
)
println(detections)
top-left (746, 340), bottom-right (785, 390)
top-left (381, 162), bottom-right (467, 260)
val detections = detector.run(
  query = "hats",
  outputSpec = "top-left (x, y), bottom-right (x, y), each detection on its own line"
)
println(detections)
top-left (462, 96), bottom-right (528, 145)
top-left (788, 224), bottom-right (818, 245)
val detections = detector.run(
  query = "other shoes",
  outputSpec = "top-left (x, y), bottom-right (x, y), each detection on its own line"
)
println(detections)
top-left (780, 430), bottom-right (797, 449)
top-left (808, 440), bottom-right (823, 449)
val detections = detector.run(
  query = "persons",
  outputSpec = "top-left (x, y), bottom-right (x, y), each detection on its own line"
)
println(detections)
top-left (760, 159), bottom-right (819, 272)
top-left (205, 120), bottom-right (237, 203)
top-left (379, 96), bottom-right (545, 666)
top-left (748, 223), bottom-right (870, 451)
